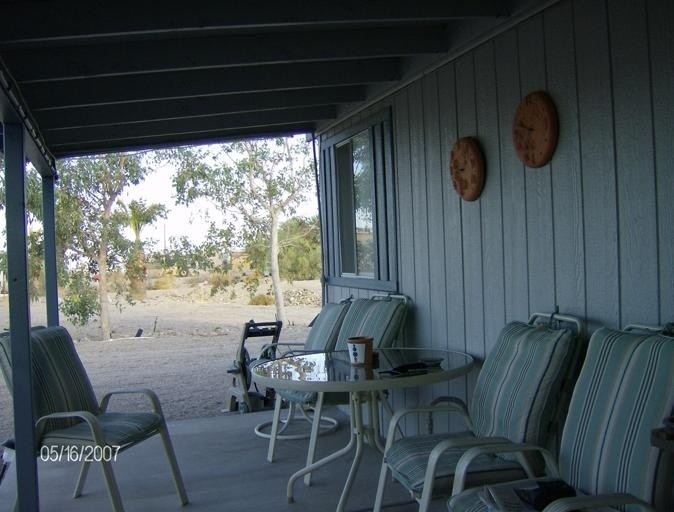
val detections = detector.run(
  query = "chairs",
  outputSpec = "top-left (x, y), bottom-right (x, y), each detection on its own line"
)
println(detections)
top-left (446, 323), bottom-right (672, 510)
top-left (372, 312), bottom-right (584, 512)
top-left (249, 294), bottom-right (409, 486)
top-left (0, 325), bottom-right (188, 511)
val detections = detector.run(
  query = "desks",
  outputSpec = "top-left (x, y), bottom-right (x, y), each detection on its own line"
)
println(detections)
top-left (250, 346), bottom-right (474, 511)
